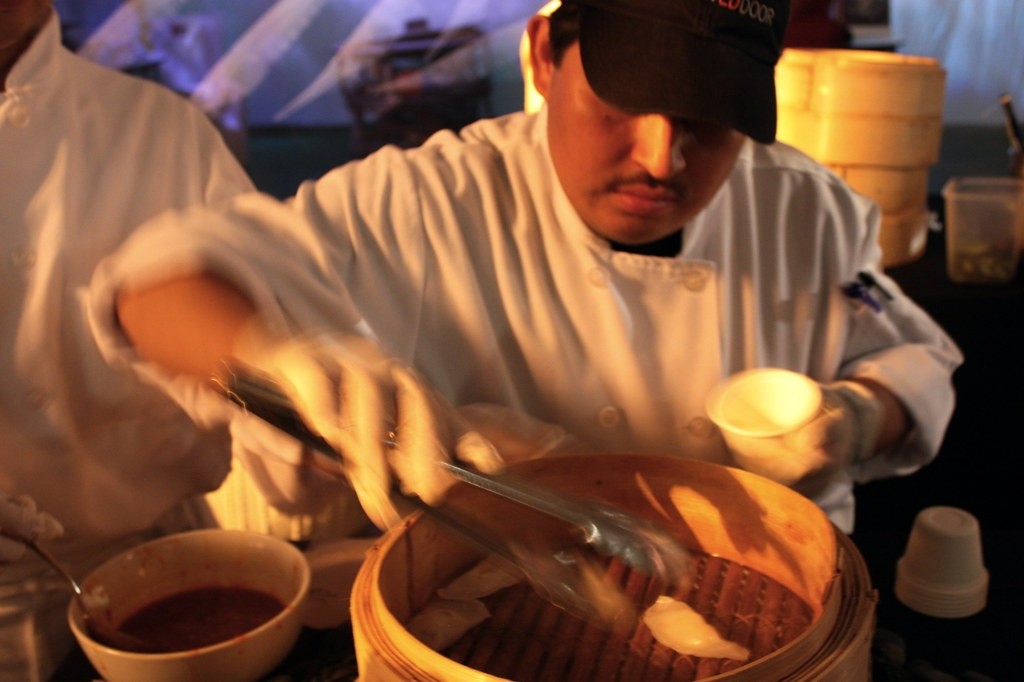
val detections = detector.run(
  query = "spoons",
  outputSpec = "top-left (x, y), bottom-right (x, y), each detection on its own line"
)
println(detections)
top-left (18, 533), bottom-right (147, 651)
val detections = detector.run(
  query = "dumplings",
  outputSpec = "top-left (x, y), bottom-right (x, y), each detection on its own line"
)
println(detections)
top-left (436, 551), bottom-right (525, 602)
top-left (402, 597), bottom-right (492, 652)
top-left (643, 593), bottom-right (749, 661)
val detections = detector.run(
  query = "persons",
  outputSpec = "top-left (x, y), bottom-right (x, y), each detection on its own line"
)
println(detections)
top-left (0, 0), bottom-right (268, 563)
top-left (85, 0), bottom-right (965, 627)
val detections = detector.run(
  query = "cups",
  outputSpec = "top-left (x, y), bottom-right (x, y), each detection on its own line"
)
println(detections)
top-left (706, 366), bottom-right (824, 489)
top-left (894, 506), bottom-right (988, 619)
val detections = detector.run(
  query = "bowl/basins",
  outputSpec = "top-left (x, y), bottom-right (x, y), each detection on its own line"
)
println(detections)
top-left (67, 529), bottom-right (312, 682)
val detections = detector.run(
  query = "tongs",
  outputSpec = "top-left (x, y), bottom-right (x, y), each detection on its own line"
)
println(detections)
top-left (215, 356), bottom-right (692, 634)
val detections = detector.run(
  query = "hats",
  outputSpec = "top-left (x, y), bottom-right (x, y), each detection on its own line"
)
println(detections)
top-left (560, 0), bottom-right (790, 145)
